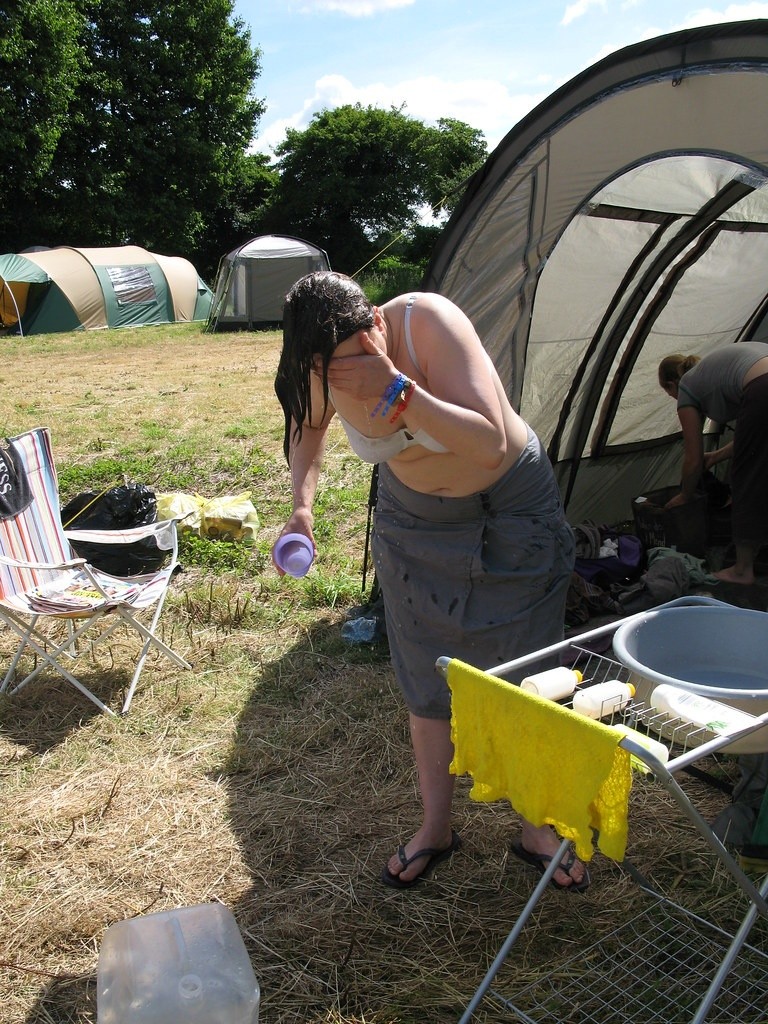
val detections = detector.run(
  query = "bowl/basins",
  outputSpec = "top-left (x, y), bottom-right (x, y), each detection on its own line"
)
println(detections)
top-left (614, 606), bottom-right (768, 752)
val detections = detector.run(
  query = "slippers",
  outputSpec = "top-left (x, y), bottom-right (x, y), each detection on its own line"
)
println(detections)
top-left (511, 835), bottom-right (591, 891)
top-left (383, 828), bottom-right (461, 889)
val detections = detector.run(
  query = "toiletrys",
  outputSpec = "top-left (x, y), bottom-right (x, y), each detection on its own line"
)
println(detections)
top-left (573, 680), bottom-right (636, 719)
top-left (650, 683), bottom-right (763, 737)
top-left (521, 667), bottom-right (582, 702)
top-left (607, 724), bottom-right (669, 764)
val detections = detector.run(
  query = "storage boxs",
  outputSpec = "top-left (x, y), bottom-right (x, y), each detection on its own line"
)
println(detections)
top-left (98, 903), bottom-right (259, 1024)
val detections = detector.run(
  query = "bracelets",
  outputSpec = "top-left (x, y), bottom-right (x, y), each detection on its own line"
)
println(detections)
top-left (369, 372), bottom-right (416, 423)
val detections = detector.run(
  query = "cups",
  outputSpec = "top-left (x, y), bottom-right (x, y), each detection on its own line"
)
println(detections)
top-left (273, 534), bottom-right (315, 577)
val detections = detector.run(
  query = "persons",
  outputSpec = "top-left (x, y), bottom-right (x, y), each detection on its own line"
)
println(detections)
top-left (268, 272), bottom-right (592, 891)
top-left (658, 339), bottom-right (768, 589)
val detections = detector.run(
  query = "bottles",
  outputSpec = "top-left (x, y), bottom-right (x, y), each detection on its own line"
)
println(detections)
top-left (96, 903), bottom-right (261, 1024)
top-left (606, 724), bottom-right (669, 765)
top-left (521, 667), bottom-right (582, 702)
top-left (573, 680), bottom-right (635, 718)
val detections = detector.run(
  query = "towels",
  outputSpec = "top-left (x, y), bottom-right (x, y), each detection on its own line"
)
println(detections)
top-left (0, 437), bottom-right (34, 520)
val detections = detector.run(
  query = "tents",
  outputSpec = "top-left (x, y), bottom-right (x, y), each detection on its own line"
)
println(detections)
top-left (357, 16), bottom-right (768, 608)
top-left (0, 245), bottom-right (221, 336)
top-left (205, 232), bottom-right (340, 340)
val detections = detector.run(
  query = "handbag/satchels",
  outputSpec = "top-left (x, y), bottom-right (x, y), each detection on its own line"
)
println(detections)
top-left (631, 485), bottom-right (706, 565)
top-left (153, 491), bottom-right (260, 544)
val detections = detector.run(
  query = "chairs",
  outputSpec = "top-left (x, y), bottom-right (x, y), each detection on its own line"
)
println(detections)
top-left (0, 426), bottom-right (193, 718)
